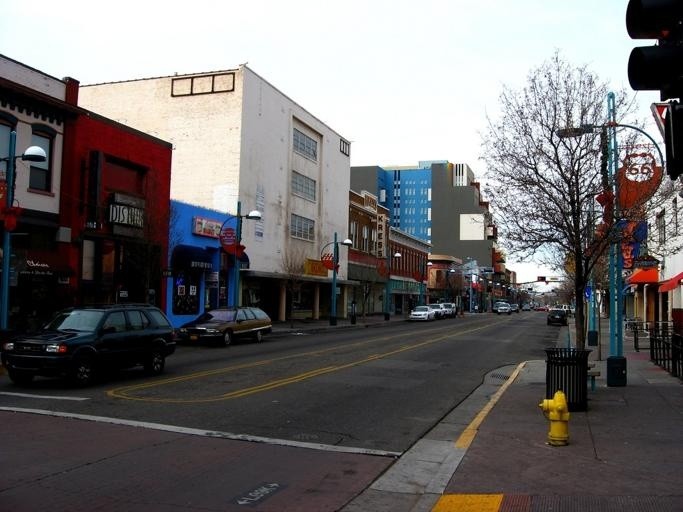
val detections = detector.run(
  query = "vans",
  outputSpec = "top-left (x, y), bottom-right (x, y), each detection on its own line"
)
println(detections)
top-left (0, 300), bottom-right (177, 389)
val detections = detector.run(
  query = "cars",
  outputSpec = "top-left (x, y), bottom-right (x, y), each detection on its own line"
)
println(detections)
top-left (407, 302), bottom-right (456, 321)
top-left (492, 301), bottom-right (575, 315)
top-left (179, 306), bottom-right (272, 348)
top-left (547, 309), bottom-right (567, 326)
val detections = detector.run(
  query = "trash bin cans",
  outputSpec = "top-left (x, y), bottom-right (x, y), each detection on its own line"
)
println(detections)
top-left (541, 347), bottom-right (594, 413)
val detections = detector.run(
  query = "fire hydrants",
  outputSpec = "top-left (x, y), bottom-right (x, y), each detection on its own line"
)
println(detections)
top-left (537, 390), bottom-right (570, 447)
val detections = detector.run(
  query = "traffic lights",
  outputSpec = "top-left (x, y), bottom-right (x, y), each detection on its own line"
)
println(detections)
top-left (626, 1), bottom-right (681, 182)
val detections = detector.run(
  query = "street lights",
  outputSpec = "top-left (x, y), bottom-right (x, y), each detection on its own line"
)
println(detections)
top-left (412, 256), bottom-right (433, 306)
top-left (218, 201), bottom-right (262, 306)
top-left (555, 92), bottom-right (664, 387)
top-left (1, 129), bottom-right (48, 355)
top-left (320, 231), bottom-right (353, 325)
top-left (376, 244), bottom-right (401, 320)
